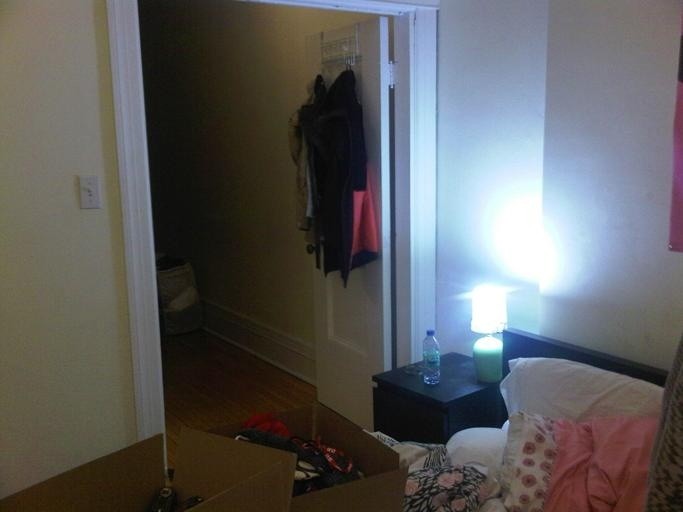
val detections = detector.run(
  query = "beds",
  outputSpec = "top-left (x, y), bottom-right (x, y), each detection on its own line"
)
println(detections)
top-left (362, 328), bottom-right (668, 512)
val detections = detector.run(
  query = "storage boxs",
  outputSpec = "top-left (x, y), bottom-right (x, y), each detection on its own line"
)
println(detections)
top-left (208, 402), bottom-right (409, 512)
top-left (0, 426), bottom-right (298, 512)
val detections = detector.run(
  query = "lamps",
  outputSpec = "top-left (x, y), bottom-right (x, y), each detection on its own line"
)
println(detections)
top-left (471, 285), bottom-right (507, 383)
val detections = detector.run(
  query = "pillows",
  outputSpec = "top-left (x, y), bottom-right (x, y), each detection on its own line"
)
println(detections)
top-left (448, 357), bottom-right (665, 512)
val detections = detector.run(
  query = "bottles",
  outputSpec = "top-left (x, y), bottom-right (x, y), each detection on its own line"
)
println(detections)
top-left (422, 330), bottom-right (440, 385)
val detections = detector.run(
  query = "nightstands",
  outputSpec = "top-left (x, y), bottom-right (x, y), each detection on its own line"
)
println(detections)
top-left (373, 352), bottom-right (503, 445)
